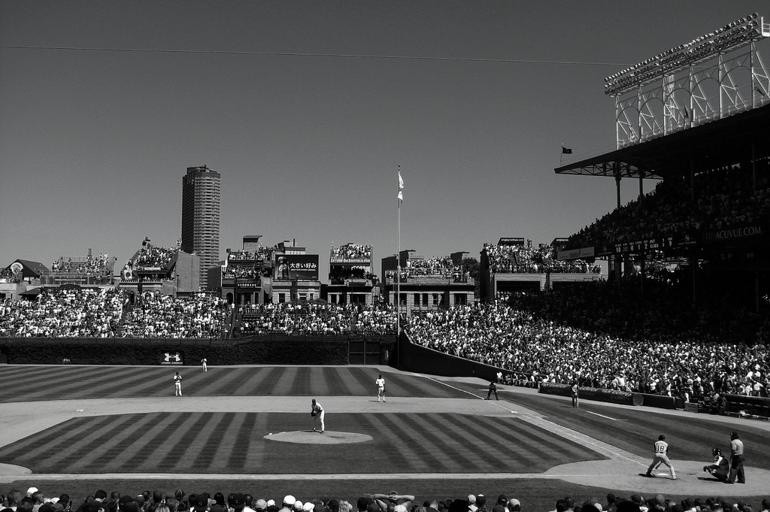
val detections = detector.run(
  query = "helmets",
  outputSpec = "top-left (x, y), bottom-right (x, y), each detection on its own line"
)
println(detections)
top-left (27, 487), bottom-right (38, 496)
top-left (244, 494), bottom-right (315, 511)
top-left (498, 494), bottom-right (520, 508)
top-left (468, 494), bottom-right (485, 504)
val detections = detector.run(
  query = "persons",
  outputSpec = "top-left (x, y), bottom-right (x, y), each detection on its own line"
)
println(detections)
top-left (311, 399), bottom-right (325, 433)
top-left (646, 434), bottom-right (677, 480)
top-left (1, 162), bottom-right (770, 416)
top-left (704, 448), bottom-right (729, 482)
top-left (0, 486), bottom-right (769, 512)
top-left (725, 431), bottom-right (745, 484)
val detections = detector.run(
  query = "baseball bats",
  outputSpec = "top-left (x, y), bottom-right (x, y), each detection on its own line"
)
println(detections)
top-left (655, 462), bottom-right (662, 469)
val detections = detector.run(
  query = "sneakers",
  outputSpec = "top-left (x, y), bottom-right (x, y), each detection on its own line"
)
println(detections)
top-left (724, 480), bottom-right (745, 483)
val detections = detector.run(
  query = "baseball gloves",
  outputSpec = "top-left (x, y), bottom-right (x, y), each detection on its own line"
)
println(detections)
top-left (703, 467), bottom-right (709, 471)
top-left (312, 413), bottom-right (316, 416)
